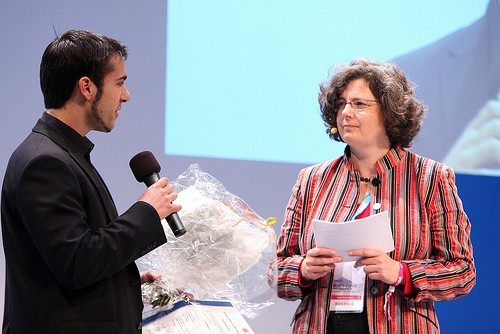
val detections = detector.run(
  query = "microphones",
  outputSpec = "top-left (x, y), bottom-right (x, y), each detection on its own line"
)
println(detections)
top-left (331, 127), bottom-right (338, 134)
top-left (129, 151), bottom-right (186, 237)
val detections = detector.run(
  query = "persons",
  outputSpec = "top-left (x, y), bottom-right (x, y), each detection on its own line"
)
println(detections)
top-left (268, 58), bottom-right (477, 334)
top-left (0, 29), bottom-right (194, 334)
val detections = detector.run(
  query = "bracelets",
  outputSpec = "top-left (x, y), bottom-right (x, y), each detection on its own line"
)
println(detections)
top-left (382, 261), bottom-right (405, 320)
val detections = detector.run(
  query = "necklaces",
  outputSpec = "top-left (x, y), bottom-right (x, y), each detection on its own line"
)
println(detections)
top-left (360, 176), bottom-right (371, 182)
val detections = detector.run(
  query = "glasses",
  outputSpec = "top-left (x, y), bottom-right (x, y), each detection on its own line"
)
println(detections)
top-left (336, 97), bottom-right (380, 112)
